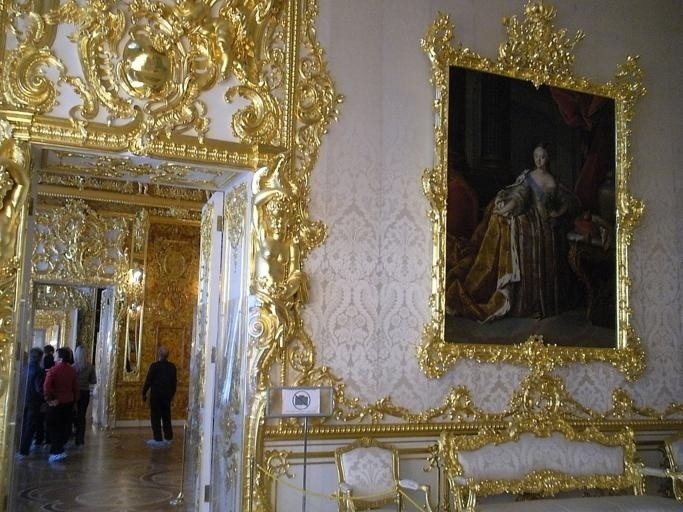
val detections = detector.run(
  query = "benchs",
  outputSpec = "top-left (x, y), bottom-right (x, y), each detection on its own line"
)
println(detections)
top-left (440, 413), bottom-right (683, 511)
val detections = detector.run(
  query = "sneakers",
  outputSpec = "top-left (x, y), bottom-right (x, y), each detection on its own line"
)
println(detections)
top-left (48, 453), bottom-right (67, 463)
top-left (147, 440), bottom-right (172, 446)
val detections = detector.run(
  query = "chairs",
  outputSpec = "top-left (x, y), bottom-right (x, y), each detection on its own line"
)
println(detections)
top-left (334, 436), bottom-right (434, 511)
top-left (664, 427), bottom-right (682, 501)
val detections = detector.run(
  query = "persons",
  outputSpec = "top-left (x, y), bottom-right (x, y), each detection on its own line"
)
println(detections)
top-left (484, 139), bottom-right (561, 321)
top-left (143, 346), bottom-right (177, 446)
top-left (15, 345), bottom-right (98, 462)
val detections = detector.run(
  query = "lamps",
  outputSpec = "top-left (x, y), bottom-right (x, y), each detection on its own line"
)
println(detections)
top-left (128, 301), bottom-right (142, 321)
top-left (128, 260), bottom-right (143, 286)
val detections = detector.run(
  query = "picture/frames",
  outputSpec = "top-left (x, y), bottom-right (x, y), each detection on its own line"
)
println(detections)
top-left (410, 0), bottom-right (649, 388)
